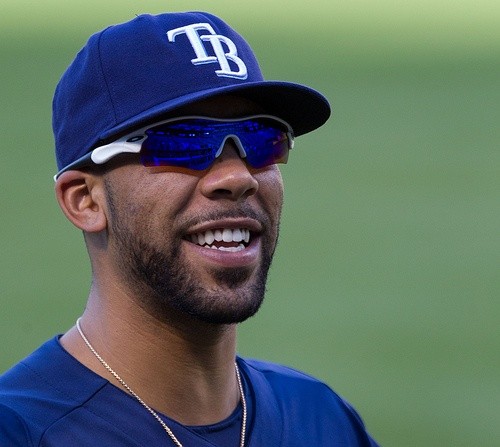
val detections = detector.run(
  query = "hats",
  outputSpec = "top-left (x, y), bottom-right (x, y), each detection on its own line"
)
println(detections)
top-left (52, 12), bottom-right (332, 171)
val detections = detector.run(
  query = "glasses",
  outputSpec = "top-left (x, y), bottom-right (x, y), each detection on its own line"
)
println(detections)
top-left (53, 114), bottom-right (295, 182)
